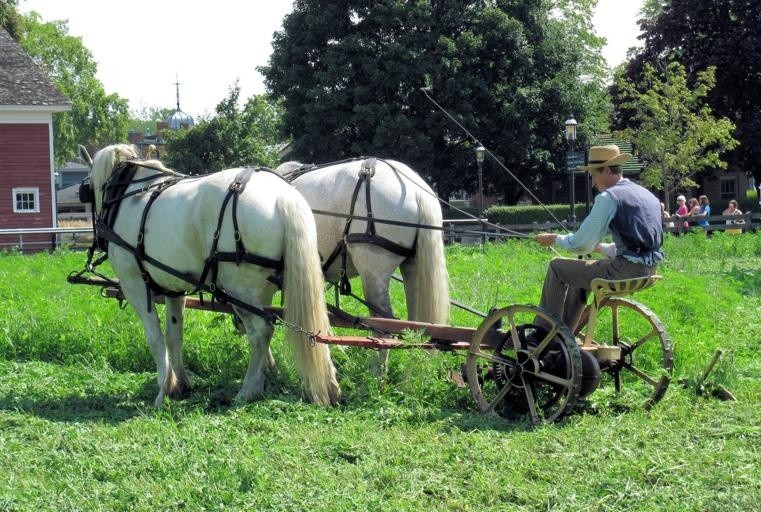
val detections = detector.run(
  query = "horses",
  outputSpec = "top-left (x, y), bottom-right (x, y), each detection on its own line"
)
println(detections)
top-left (78, 145), bottom-right (343, 408)
top-left (231, 159), bottom-right (450, 392)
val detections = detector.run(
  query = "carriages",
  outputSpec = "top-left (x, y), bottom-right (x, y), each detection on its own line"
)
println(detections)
top-left (65, 139), bottom-right (677, 431)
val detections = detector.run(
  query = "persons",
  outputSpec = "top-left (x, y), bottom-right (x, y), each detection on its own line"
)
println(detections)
top-left (660, 202), bottom-right (673, 227)
top-left (722, 200), bottom-right (744, 234)
top-left (524, 144), bottom-right (662, 351)
top-left (676, 198), bottom-right (699, 226)
top-left (672, 195), bottom-right (688, 237)
top-left (696, 195), bottom-right (710, 235)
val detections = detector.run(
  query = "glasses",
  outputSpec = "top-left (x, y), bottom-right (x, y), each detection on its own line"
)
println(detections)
top-left (675, 200), bottom-right (683, 202)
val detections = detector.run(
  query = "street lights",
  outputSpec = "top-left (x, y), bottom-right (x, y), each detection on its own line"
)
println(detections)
top-left (562, 113), bottom-right (582, 224)
top-left (472, 141), bottom-right (489, 252)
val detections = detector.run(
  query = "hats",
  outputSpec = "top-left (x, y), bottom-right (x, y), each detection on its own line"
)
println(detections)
top-left (575, 143), bottom-right (632, 171)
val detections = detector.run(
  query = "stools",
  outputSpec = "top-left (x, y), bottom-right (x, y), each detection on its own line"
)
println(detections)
top-left (583, 275), bottom-right (665, 347)
